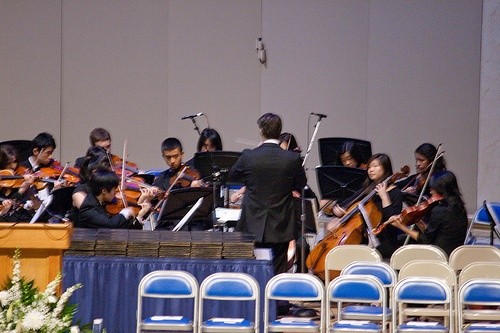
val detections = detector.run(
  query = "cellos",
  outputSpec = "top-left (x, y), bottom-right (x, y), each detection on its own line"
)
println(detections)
top-left (304, 164), bottom-right (411, 282)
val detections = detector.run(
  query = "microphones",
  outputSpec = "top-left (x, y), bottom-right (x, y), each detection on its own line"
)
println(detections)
top-left (181, 112), bottom-right (203, 120)
top-left (310, 113), bottom-right (328, 118)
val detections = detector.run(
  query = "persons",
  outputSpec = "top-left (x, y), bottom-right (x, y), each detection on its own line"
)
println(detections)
top-left (230, 111), bottom-right (308, 314)
top-left (332, 154), bottom-right (403, 254)
top-left (319, 142), bottom-right (366, 217)
top-left (388, 169), bottom-right (468, 256)
top-left (150, 137), bottom-right (211, 232)
top-left (184, 128), bottom-right (224, 168)
top-left (395, 142), bottom-right (446, 199)
top-left (0, 127), bottom-right (159, 231)
top-left (277, 133), bottom-right (301, 155)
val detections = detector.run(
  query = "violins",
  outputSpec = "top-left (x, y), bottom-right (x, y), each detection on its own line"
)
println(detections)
top-left (371, 192), bottom-right (447, 236)
top-left (0, 152), bottom-right (201, 222)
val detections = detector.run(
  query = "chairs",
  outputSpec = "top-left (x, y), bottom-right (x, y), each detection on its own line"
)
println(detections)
top-left (457, 277), bottom-right (500, 333)
top-left (458, 261), bottom-right (500, 332)
top-left (447, 244), bottom-right (500, 275)
top-left (325, 244), bottom-right (383, 333)
top-left (263, 272), bottom-right (327, 333)
top-left (388, 244), bottom-right (449, 332)
top-left (135, 269), bottom-right (200, 333)
top-left (337, 260), bottom-right (398, 333)
top-left (198, 271), bottom-right (261, 333)
top-left (391, 276), bottom-right (455, 332)
top-left (462, 202), bottom-right (500, 248)
top-left (325, 275), bottom-right (388, 333)
top-left (397, 259), bottom-right (458, 333)
